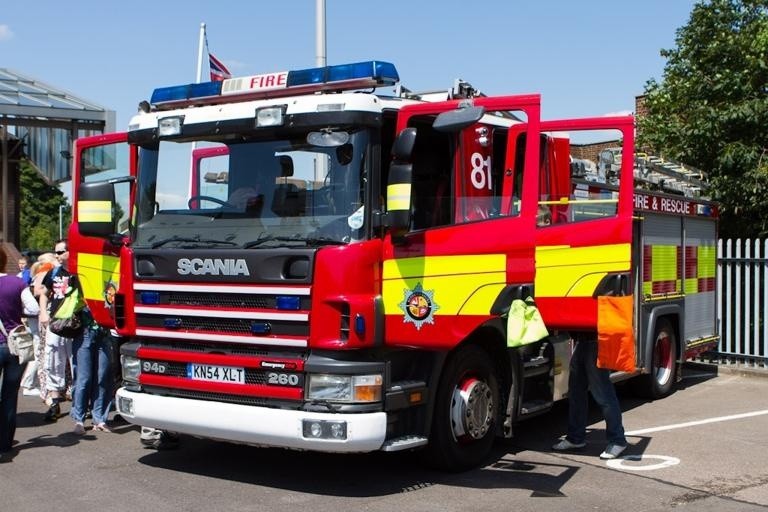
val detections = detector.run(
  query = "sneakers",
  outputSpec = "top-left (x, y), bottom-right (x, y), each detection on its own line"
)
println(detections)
top-left (599, 444), bottom-right (627, 459)
top-left (552, 439), bottom-right (586, 451)
top-left (44, 404), bottom-right (60, 421)
top-left (93, 422), bottom-right (110, 433)
top-left (74, 422), bottom-right (85, 435)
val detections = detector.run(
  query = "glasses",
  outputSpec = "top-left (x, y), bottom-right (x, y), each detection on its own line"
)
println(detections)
top-left (56, 251), bottom-right (65, 255)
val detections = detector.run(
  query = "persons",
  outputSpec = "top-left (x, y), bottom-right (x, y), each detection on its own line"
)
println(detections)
top-left (0, 250), bottom-right (42, 455)
top-left (548, 330), bottom-right (629, 460)
top-left (14, 238), bottom-right (129, 438)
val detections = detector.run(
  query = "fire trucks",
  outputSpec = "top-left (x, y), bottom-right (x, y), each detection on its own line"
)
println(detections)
top-left (56, 60), bottom-right (722, 472)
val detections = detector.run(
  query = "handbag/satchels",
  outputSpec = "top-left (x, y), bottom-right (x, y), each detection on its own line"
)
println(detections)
top-left (8, 325), bottom-right (35, 365)
top-left (50, 288), bottom-right (85, 337)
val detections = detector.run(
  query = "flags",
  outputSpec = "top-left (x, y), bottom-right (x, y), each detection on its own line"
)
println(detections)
top-left (209, 54), bottom-right (232, 81)
